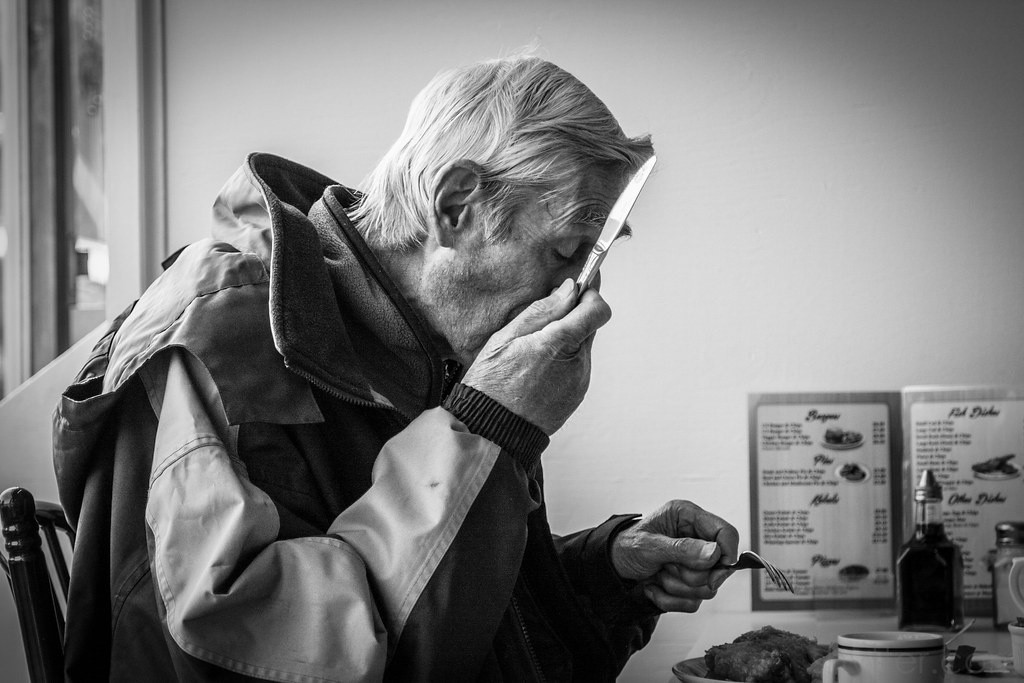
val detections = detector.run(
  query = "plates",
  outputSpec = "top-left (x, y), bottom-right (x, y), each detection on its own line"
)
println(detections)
top-left (672, 657), bottom-right (747, 683)
top-left (972, 461), bottom-right (1021, 479)
top-left (835, 465), bottom-right (869, 484)
top-left (820, 433), bottom-right (865, 449)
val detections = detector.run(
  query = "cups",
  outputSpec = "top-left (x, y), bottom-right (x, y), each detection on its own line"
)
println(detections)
top-left (823, 632), bottom-right (945, 683)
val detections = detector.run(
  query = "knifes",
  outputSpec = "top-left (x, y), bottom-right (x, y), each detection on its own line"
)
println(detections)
top-left (578, 153), bottom-right (657, 296)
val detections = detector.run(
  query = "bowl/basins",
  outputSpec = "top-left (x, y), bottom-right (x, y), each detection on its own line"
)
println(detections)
top-left (1008, 623), bottom-right (1024, 677)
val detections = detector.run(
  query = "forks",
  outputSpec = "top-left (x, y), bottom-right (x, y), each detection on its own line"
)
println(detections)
top-left (716, 550), bottom-right (795, 593)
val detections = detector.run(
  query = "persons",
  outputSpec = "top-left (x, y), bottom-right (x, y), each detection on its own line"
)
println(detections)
top-left (49, 55), bottom-right (739, 683)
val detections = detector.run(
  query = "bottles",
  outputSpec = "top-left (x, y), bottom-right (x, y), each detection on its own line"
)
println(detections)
top-left (896, 469), bottom-right (966, 633)
top-left (993, 522), bottom-right (1024, 629)
